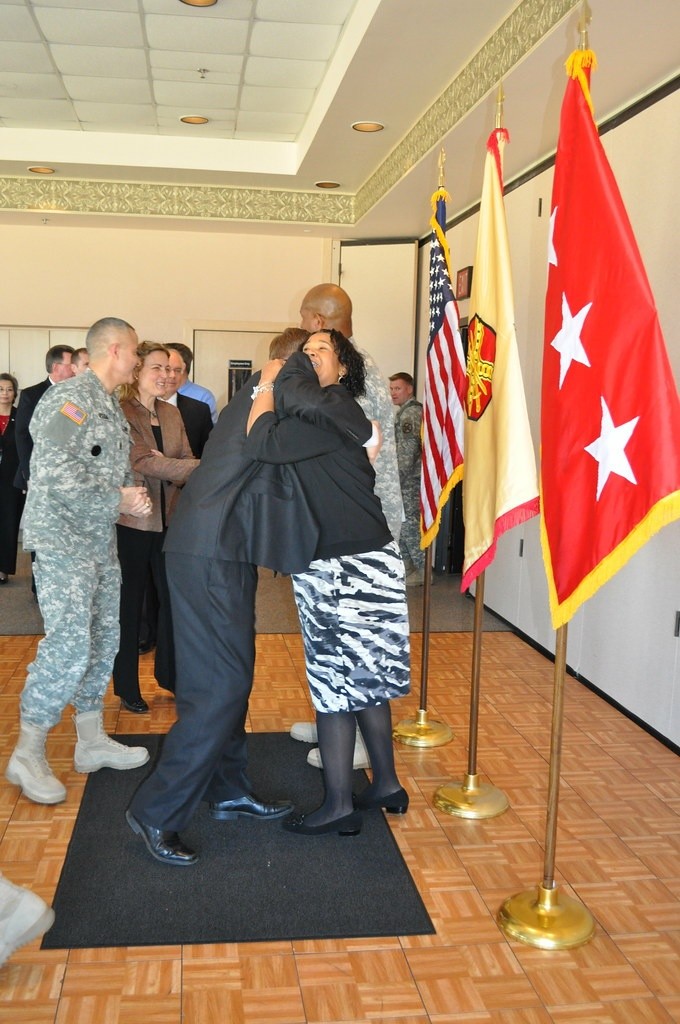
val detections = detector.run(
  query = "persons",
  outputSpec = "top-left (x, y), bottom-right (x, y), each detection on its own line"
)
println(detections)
top-left (112, 342), bottom-right (225, 713)
top-left (0, 345), bottom-right (100, 596)
top-left (126, 328), bottom-right (383, 866)
top-left (244, 328), bottom-right (410, 837)
top-left (289, 282), bottom-right (407, 771)
top-left (5, 317), bottom-right (155, 805)
top-left (388, 372), bottom-right (434, 587)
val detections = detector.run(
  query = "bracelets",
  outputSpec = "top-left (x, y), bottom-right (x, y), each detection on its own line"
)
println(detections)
top-left (251, 382), bottom-right (274, 400)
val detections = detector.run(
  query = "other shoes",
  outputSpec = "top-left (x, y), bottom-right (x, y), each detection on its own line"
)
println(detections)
top-left (34, 594), bottom-right (39, 603)
top-left (307, 728), bottom-right (372, 771)
top-left (0, 572), bottom-right (8, 585)
top-left (0, 874), bottom-right (56, 969)
top-left (289, 722), bottom-right (318, 744)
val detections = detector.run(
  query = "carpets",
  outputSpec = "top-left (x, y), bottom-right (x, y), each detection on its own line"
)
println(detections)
top-left (40, 731), bottom-right (436, 952)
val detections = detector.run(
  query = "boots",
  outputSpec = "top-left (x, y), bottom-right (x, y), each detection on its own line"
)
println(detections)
top-left (4, 719), bottom-right (67, 804)
top-left (70, 709), bottom-right (150, 774)
top-left (404, 566), bottom-right (434, 586)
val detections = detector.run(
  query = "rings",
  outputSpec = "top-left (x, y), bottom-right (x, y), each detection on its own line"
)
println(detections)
top-left (145, 503), bottom-right (150, 507)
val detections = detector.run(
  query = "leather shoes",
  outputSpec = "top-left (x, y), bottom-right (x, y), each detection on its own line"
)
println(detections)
top-left (206, 790), bottom-right (294, 820)
top-left (139, 637), bottom-right (156, 655)
top-left (158, 682), bottom-right (178, 695)
top-left (126, 808), bottom-right (199, 865)
top-left (120, 697), bottom-right (148, 714)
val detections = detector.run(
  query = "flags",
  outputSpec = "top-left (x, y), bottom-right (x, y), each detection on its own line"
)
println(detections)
top-left (460, 127), bottom-right (539, 595)
top-left (539, 49), bottom-right (680, 631)
top-left (419, 185), bottom-right (467, 552)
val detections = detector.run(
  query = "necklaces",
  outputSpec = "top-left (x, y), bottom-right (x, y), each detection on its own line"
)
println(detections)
top-left (152, 410), bottom-right (158, 417)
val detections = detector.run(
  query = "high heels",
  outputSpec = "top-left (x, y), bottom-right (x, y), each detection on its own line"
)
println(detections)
top-left (281, 805), bottom-right (363, 838)
top-left (350, 785), bottom-right (410, 813)
